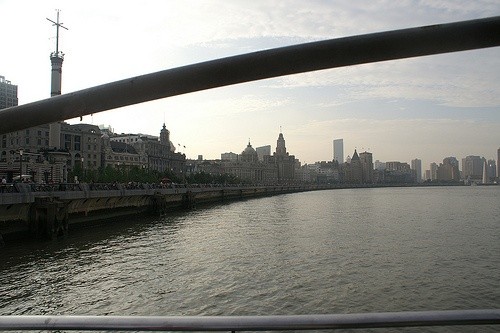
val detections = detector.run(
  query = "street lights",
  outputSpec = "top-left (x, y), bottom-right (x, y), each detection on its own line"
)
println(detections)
top-left (19, 145), bottom-right (24, 183)
top-left (81, 155), bottom-right (84, 182)
top-left (117, 160), bottom-right (120, 183)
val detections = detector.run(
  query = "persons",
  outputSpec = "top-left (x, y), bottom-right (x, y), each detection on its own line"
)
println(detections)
top-left (1, 176), bottom-right (9, 192)
